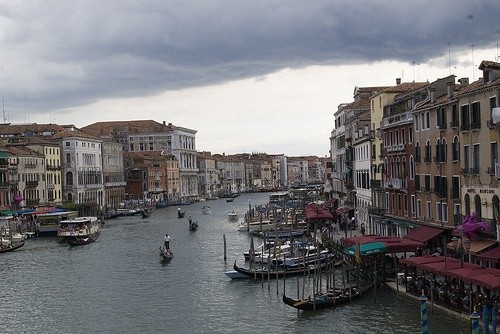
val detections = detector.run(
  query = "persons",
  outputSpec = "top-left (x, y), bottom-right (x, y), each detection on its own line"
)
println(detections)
top-left (177, 207), bottom-right (181, 213)
top-left (164, 234), bottom-right (171, 252)
top-left (187, 215), bottom-right (196, 228)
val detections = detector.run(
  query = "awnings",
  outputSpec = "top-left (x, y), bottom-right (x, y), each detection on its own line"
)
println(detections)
top-left (305, 198), bottom-right (353, 220)
top-left (342, 226), bottom-right (500, 290)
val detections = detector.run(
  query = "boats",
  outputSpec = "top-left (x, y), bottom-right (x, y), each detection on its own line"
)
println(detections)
top-left (228, 212), bottom-right (239, 220)
top-left (157, 194), bottom-right (221, 208)
top-left (189, 221), bottom-right (198, 231)
top-left (56, 216), bottom-right (102, 245)
top-left (201, 208), bottom-right (210, 215)
top-left (159, 246), bottom-right (174, 259)
top-left (233, 184), bottom-right (500, 330)
top-left (177, 211), bottom-right (186, 218)
top-left (226, 198), bottom-right (234, 202)
top-left (0, 204), bottom-right (157, 252)
